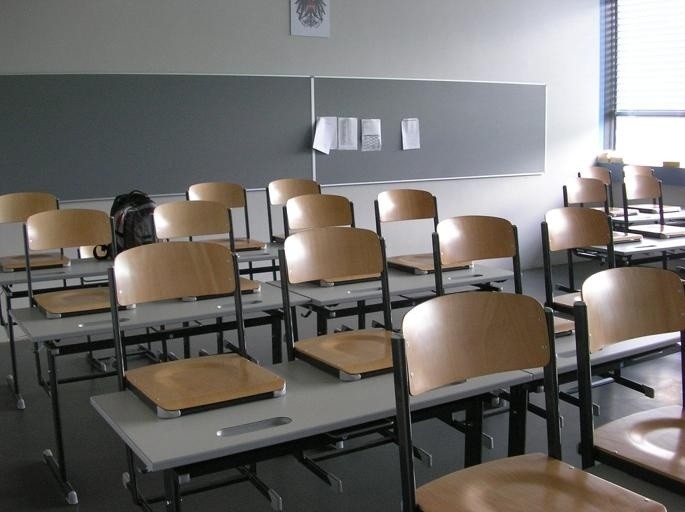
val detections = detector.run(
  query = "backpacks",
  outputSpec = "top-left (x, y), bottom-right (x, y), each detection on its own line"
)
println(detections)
top-left (93, 189), bottom-right (156, 260)
